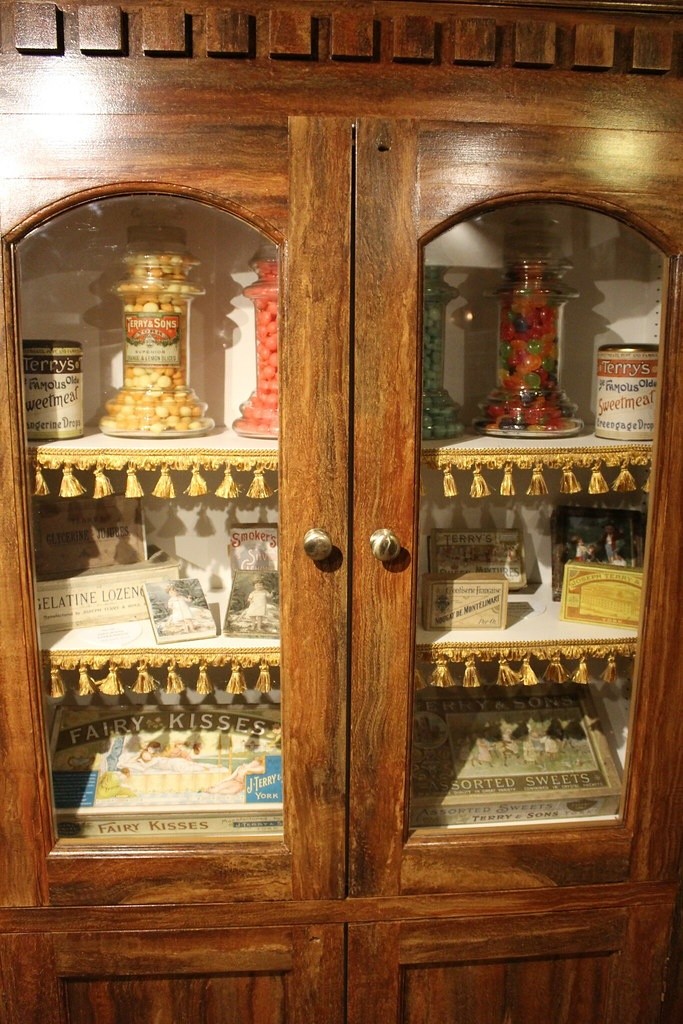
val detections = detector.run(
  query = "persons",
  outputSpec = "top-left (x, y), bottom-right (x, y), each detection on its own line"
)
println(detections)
top-left (247, 580), bottom-right (276, 632)
top-left (563, 519), bottom-right (632, 569)
top-left (167, 585), bottom-right (199, 634)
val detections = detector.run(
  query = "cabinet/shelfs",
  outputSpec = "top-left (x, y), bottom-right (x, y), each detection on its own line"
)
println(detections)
top-left (0, 0), bottom-right (683, 1024)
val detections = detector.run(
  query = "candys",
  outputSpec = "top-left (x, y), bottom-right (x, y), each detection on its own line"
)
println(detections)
top-left (421, 265), bottom-right (465, 439)
top-left (236, 257), bottom-right (279, 436)
top-left (478, 250), bottom-right (578, 432)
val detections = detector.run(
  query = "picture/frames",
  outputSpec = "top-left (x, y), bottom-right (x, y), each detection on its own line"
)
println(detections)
top-left (550, 504), bottom-right (641, 601)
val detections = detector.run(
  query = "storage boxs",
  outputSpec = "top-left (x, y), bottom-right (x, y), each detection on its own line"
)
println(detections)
top-left (426, 528), bottom-right (526, 591)
top-left (51, 700), bottom-right (286, 845)
top-left (37, 546), bottom-right (179, 638)
top-left (407, 679), bottom-right (621, 827)
top-left (424, 573), bottom-right (507, 630)
top-left (563, 561), bottom-right (644, 629)
